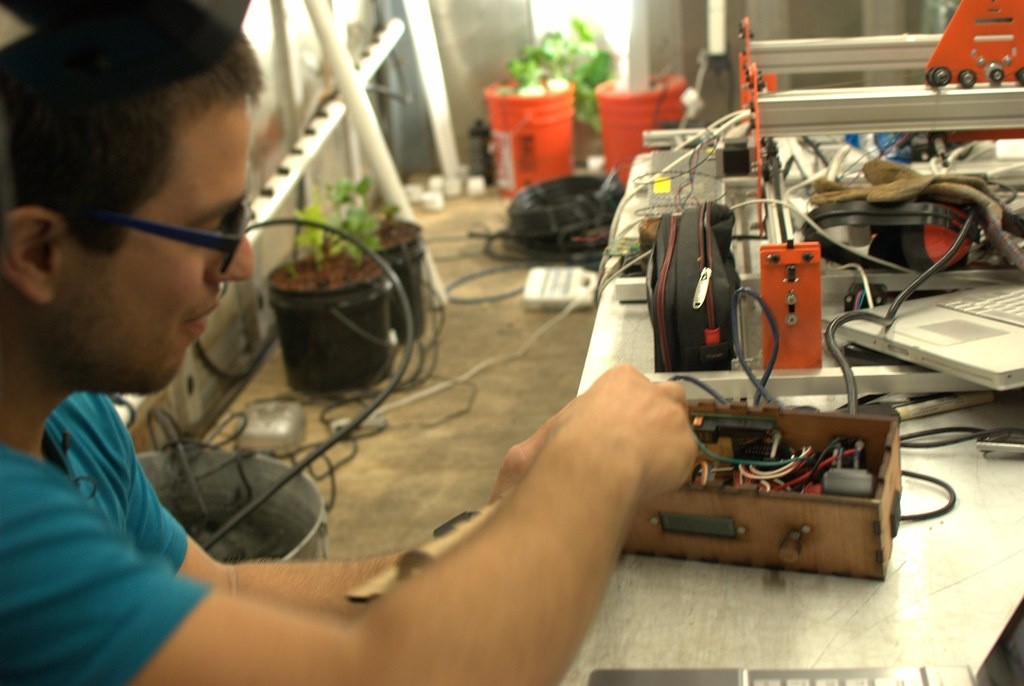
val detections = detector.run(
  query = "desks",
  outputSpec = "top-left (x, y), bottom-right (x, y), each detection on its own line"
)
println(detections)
top-left (553, 144), bottom-right (1023, 686)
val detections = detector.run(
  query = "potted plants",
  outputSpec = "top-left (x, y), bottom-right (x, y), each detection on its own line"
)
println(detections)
top-left (485, 20), bottom-right (617, 199)
top-left (312, 179), bottom-right (424, 343)
top-left (269, 199), bottom-right (397, 391)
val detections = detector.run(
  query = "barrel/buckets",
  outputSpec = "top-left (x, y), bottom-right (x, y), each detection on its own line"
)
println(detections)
top-left (594, 75), bottom-right (687, 184)
top-left (134, 448), bottom-right (331, 562)
top-left (483, 77), bottom-right (574, 197)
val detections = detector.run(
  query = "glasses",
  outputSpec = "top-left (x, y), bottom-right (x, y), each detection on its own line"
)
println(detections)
top-left (122, 191), bottom-right (254, 272)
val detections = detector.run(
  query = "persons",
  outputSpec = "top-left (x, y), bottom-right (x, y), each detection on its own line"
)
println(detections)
top-left (3, 0), bottom-right (708, 682)
